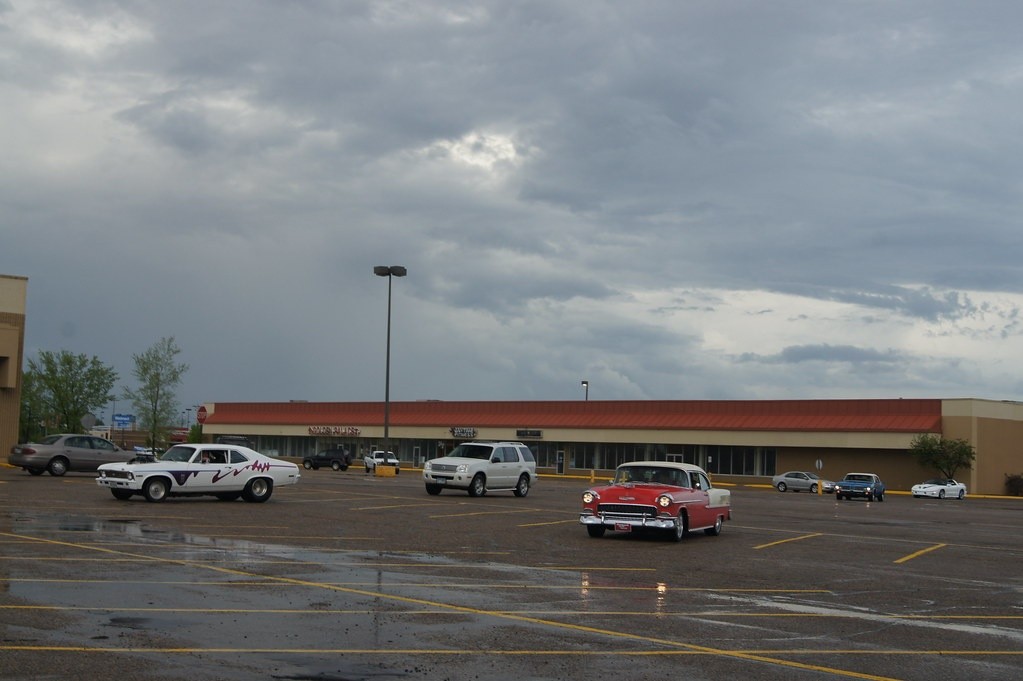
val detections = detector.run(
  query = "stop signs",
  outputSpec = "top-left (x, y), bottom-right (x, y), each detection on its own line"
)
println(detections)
top-left (197, 406), bottom-right (207, 424)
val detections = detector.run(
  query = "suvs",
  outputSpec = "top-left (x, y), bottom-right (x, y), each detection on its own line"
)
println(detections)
top-left (422, 440), bottom-right (538, 498)
top-left (302, 449), bottom-right (353, 471)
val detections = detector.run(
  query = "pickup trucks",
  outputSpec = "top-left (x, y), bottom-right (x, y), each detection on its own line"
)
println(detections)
top-left (364, 451), bottom-right (400, 474)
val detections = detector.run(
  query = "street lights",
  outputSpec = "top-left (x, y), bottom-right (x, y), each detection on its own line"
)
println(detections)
top-left (181, 412), bottom-right (185, 427)
top-left (186, 409), bottom-right (191, 431)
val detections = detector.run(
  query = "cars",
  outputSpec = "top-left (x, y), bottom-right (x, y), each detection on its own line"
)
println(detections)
top-left (772, 471), bottom-right (837, 494)
top-left (95, 444), bottom-right (301, 503)
top-left (579, 462), bottom-right (732, 542)
top-left (911, 479), bottom-right (968, 500)
top-left (834, 473), bottom-right (885, 502)
top-left (9, 434), bottom-right (146, 477)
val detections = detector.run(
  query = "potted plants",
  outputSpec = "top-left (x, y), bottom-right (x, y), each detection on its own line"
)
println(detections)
top-left (1004, 473), bottom-right (1023, 496)
